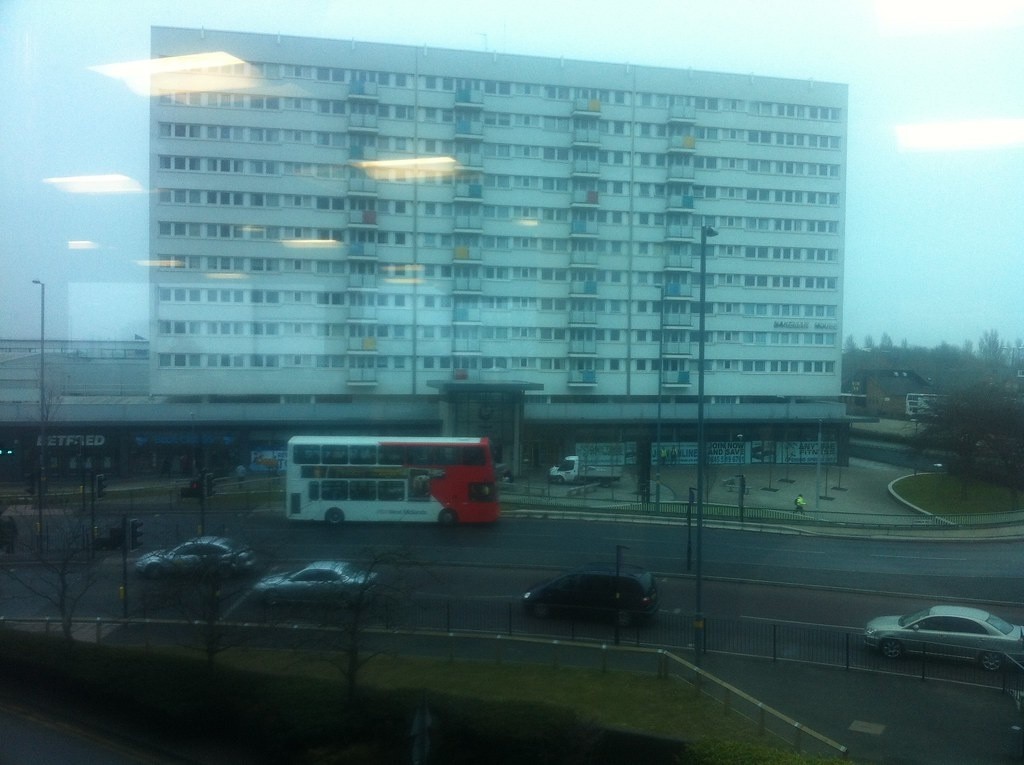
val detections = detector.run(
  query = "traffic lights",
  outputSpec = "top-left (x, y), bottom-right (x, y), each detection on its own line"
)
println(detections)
top-left (129, 518), bottom-right (145, 552)
top-left (205, 473), bottom-right (218, 497)
top-left (191, 478), bottom-right (203, 498)
top-left (96, 474), bottom-right (107, 502)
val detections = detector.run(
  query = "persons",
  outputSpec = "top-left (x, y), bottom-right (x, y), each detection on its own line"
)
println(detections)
top-left (660, 447), bottom-right (667, 464)
top-left (670, 448), bottom-right (676, 464)
top-left (1, 516), bottom-right (18, 553)
top-left (236, 463), bottom-right (246, 488)
top-left (793, 495), bottom-right (807, 515)
top-left (503, 469), bottom-right (512, 483)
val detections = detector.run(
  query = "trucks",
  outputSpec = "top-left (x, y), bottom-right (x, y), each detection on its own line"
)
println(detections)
top-left (547, 455), bottom-right (625, 487)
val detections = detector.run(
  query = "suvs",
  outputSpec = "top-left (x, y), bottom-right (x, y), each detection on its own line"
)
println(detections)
top-left (521, 561), bottom-right (662, 629)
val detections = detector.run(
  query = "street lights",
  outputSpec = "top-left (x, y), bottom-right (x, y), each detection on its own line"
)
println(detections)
top-left (614, 544), bottom-right (632, 644)
top-left (687, 487), bottom-right (701, 573)
top-left (30, 279), bottom-right (48, 494)
top-left (909, 418), bottom-right (918, 475)
top-left (737, 433), bottom-right (743, 474)
top-left (695, 224), bottom-right (719, 496)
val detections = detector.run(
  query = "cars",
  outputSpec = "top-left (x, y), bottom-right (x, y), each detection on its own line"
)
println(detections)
top-left (864, 605), bottom-right (1024, 672)
top-left (134, 535), bottom-right (256, 581)
top-left (252, 558), bottom-right (379, 610)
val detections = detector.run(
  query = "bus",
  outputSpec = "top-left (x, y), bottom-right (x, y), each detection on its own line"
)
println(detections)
top-left (283, 434), bottom-right (501, 527)
